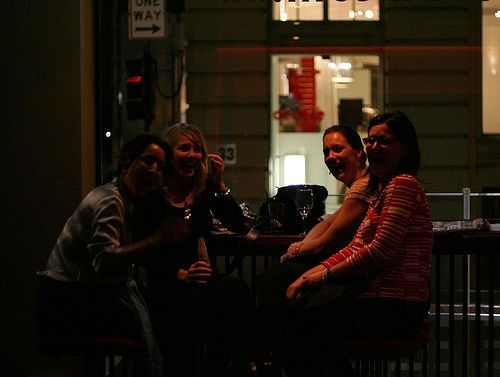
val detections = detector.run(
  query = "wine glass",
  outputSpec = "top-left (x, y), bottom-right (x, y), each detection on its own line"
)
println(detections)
top-left (294, 189), bottom-right (314, 235)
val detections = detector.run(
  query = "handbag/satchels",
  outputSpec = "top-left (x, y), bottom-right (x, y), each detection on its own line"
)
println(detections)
top-left (290, 266), bottom-right (370, 322)
top-left (278, 257), bottom-right (316, 287)
top-left (255, 185), bottom-right (328, 233)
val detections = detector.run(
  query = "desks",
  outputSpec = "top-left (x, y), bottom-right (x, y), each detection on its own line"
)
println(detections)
top-left (205, 234), bottom-right (304, 377)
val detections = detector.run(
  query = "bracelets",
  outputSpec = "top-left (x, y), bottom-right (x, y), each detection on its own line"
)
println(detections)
top-left (214, 188), bottom-right (231, 198)
top-left (321, 268), bottom-right (328, 279)
top-left (293, 242), bottom-right (303, 256)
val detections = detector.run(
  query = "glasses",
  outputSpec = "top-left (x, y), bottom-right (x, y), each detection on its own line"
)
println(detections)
top-left (362, 136), bottom-right (398, 145)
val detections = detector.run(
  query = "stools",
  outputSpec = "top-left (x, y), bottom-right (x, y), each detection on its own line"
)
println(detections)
top-left (35, 335), bottom-right (141, 377)
top-left (351, 316), bottom-right (431, 377)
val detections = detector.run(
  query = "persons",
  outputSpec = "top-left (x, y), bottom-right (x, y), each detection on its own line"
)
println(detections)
top-left (278, 112), bottom-right (432, 377)
top-left (151, 124), bottom-right (256, 376)
top-left (36, 134), bottom-right (190, 377)
top-left (256, 125), bottom-right (382, 377)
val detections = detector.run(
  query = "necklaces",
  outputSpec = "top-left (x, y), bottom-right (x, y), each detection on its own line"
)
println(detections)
top-left (183, 200), bottom-right (192, 221)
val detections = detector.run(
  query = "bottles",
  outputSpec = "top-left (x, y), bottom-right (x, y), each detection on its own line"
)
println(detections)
top-left (198, 238), bottom-right (211, 269)
